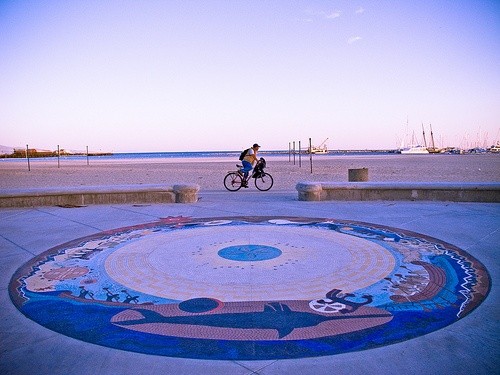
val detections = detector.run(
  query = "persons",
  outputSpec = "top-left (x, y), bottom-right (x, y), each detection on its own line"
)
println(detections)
top-left (238, 144), bottom-right (261, 188)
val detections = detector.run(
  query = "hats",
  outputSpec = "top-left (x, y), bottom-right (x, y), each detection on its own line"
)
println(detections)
top-left (253, 144), bottom-right (260, 147)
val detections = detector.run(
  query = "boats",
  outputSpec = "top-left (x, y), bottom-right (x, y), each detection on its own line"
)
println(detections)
top-left (303, 138), bottom-right (329, 155)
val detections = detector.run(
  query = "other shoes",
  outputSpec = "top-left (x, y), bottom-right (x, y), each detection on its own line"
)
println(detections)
top-left (238, 169), bottom-right (243, 176)
top-left (241, 182), bottom-right (248, 187)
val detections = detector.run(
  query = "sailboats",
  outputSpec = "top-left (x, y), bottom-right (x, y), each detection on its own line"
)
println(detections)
top-left (393, 117), bottom-right (500, 156)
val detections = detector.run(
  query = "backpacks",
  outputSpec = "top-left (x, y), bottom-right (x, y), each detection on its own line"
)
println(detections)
top-left (239, 148), bottom-right (255, 160)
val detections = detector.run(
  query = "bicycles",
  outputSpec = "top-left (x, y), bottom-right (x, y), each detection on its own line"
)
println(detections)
top-left (223, 160), bottom-right (273, 192)
